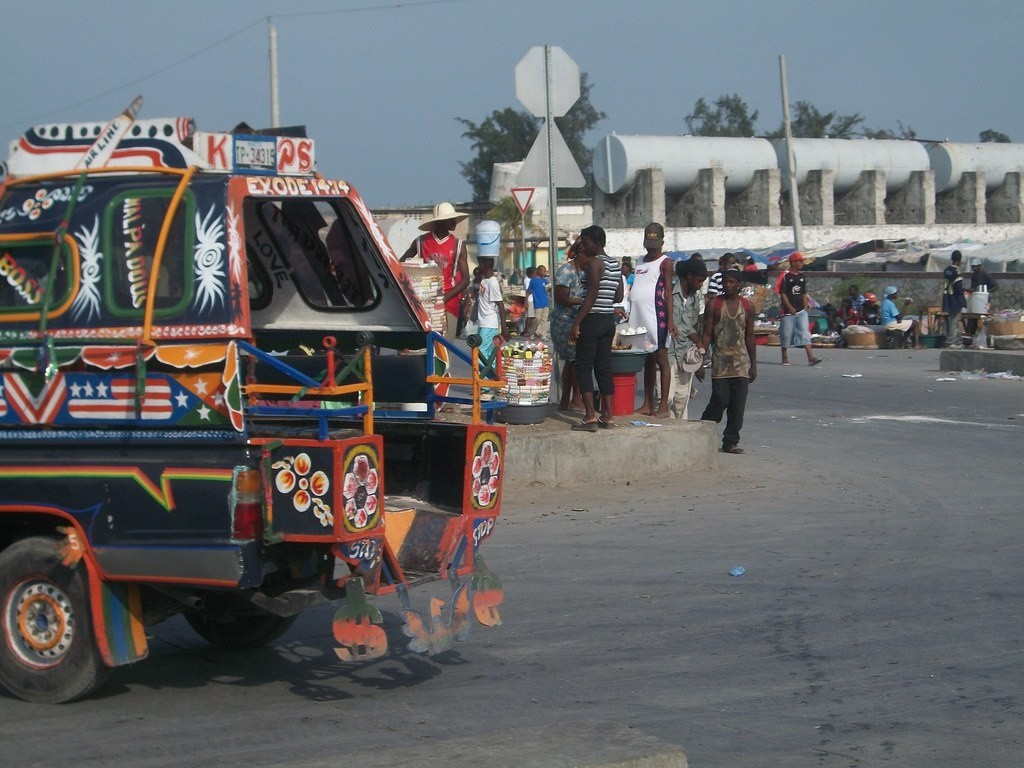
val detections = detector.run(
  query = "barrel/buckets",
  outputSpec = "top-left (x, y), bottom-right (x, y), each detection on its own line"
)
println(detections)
top-left (599, 373), bottom-right (636, 415)
top-left (619, 330), bottom-right (648, 350)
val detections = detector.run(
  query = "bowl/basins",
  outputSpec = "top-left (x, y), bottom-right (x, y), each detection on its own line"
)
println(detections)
top-left (909, 334), bottom-right (945, 348)
top-left (494, 397), bottom-right (553, 425)
top-left (610, 349), bottom-right (650, 374)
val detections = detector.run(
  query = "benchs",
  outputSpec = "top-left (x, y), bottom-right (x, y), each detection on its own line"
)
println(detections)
top-left (237, 328), bottom-right (509, 544)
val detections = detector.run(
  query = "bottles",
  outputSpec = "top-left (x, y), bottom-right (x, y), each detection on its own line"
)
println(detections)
top-left (500, 340), bottom-right (551, 359)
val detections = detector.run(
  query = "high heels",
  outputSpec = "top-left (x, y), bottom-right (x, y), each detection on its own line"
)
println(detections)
top-left (570, 420), bottom-right (597, 431)
top-left (595, 416), bottom-right (615, 430)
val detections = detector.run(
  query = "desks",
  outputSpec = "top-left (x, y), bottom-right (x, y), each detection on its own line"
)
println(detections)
top-left (753, 326), bottom-right (779, 336)
top-left (917, 305), bottom-right (994, 336)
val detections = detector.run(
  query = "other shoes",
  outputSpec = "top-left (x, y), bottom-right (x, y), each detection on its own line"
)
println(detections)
top-left (520, 329), bottom-right (528, 336)
top-left (469, 388), bottom-right (488, 395)
top-left (529, 328), bottom-right (534, 340)
top-left (702, 362), bottom-right (713, 368)
top-left (723, 443), bottom-right (743, 453)
top-left (539, 336), bottom-right (549, 341)
top-left (947, 343), bottom-right (965, 349)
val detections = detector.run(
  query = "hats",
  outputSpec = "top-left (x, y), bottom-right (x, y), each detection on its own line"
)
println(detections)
top-left (643, 222), bottom-right (665, 249)
top-left (722, 266), bottom-right (741, 279)
top-left (683, 346), bottom-right (703, 372)
top-left (789, 253), bottom-right (805, 262)
top-left (864, 293), bottom-right (876, 304)
top-left (969, 258), bottom-right (982, 265)
top-left (417, 202), bottom-right (470, 232)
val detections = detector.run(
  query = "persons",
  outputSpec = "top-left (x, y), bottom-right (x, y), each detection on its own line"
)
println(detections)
top-left (654, 258), bottom-right (708, 420)
top-left (689, 253), bottom-right (710, 397)
top-left (838, 299), bottom-right (859, 326)
top-left (475, 256), bottom-right (512, 396)
top-left (551, 238), bottom-right (586, 413)
top-left (861, 292), bottom-right (881, 326)
top-left (968, 258), bottom-right (998, 350)
top-left (398, 203), bottom-right (470, 341)
top-left (848, 285), bottom-right (865, 310)
top-left (942, 250), bottom-right (967, 349)
top-left (627, 223), bottom-right (678, 421)
top-left (702, 253), bottom-right (736, 369)
top-left (775, 253), bottom-right (822, 365)
top-left (695, 265), bottom-right (757, 454)
top-left (470, 265), bottom-right (550, 342)
top-left (569, 225), bottom-right (624, 432)
top-left (621, 263), bottom-right (634, 292)
top-left (881, 286), bottom-right (927, 350)
top-left (744, 256), bottom-right (758, 271)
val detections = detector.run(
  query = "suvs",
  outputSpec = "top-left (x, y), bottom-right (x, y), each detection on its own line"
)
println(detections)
top-left (1, 92), bottom-right (512, 706)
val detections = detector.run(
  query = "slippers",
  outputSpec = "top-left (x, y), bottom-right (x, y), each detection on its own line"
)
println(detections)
top-left (809, 357), bottom-right (822, 366)
top-left (782, 361), bottom-right (790, 366)
